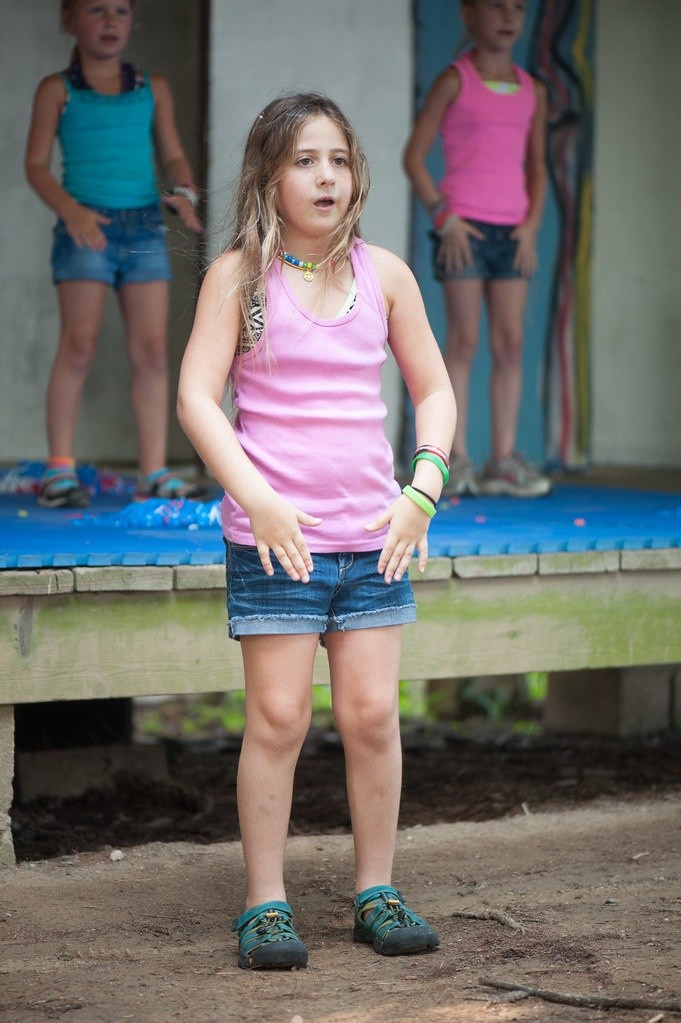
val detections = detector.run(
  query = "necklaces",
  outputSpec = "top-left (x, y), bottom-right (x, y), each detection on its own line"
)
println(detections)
top-left (277, 247), bottom-right (337, 283)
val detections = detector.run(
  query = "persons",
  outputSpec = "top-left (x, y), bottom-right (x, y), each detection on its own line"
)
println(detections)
top-left (403, 1), bottom-right (554, 498)
top-left (173, 92), bottom-right (459, 972)
top-left (22, 1), bottom-right (209, 511)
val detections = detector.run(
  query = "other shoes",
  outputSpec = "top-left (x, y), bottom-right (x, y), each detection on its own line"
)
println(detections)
top-left (36, 467), bottom-right (88, 508)
top-left (441, 452), bottom-right (478, 495)
top-left (480, 453), bottom-right (552, 496)
top-left (351, 885), bottom-right (439, 955)
top-left (131, 472), bottom-right (203, 502)
top-left (231, 901), bottom-right (308, 971)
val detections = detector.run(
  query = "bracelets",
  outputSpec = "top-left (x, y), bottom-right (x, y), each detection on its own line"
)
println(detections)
top-left (402, 485), bottom-right (437, 518)
top-left (411, 485), bottom-right (437, 507)
top-left (425, 192), bottom-right (458, 236)
top-left (170, 181), bottom-right (199, 207)
top-left (412, 444), bottom-right (450, 470)
top-left (412, 452), bottom-right (449, 487)
